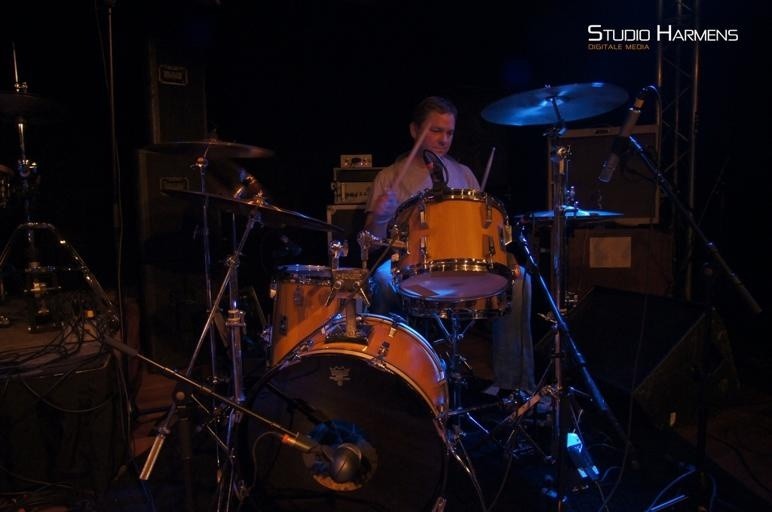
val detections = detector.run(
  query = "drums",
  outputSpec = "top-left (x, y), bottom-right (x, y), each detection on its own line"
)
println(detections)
top-left (388, 188), bottom-right (514, 292)
top-left (398, 273), bottom-right (510, 320)
top-left (246, 315), bottom-right (450, 510)
top-left (272, 266), bottom-right (368, 368)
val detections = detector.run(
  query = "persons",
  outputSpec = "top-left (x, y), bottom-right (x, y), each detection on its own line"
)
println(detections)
top-left (363, 96), bottom-right (484, 266)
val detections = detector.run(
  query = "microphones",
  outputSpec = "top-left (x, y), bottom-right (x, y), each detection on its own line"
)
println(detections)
top-left (598, 88), bottom-right (650, 182)
top-left (513, 210), bottom-right (531, 232)
top-left (422, 155), bottom-right (441, 184)
top-left (280, 433), bottom-right (361, 483)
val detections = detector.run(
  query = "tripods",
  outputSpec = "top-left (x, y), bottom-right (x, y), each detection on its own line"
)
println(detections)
top-left (446, 319), bottom-right (520, 462)
top-left (453, 144), bottom-right (618, 512)
top-left (0, 40), bottom-right (122, 334)
top-left (628, 135), bottom-right (741, 512)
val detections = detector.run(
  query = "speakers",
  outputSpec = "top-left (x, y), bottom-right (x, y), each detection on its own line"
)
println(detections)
top-left (148, 37), bottom-right (209, 146)
top-left (547, 124), bottom-right (660, 226)
top-left (534, 285), bottom-right (746, 442)
top-left (549, 221), bottom-right (687, 299)
top-left (137, 145), bottom-right (223, 334)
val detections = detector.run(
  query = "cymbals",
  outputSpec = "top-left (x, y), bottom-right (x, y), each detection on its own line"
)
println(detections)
top-left (171, 190), bottom-right (347, 232)
top-left (479, 83), bottom-right (628, 126)
top-left (514, 210), bottom-right (622, 216)
top-left (171, 142), bottom-right (269, 157)
top-left (1, 94), bottom-right (55, 123)
